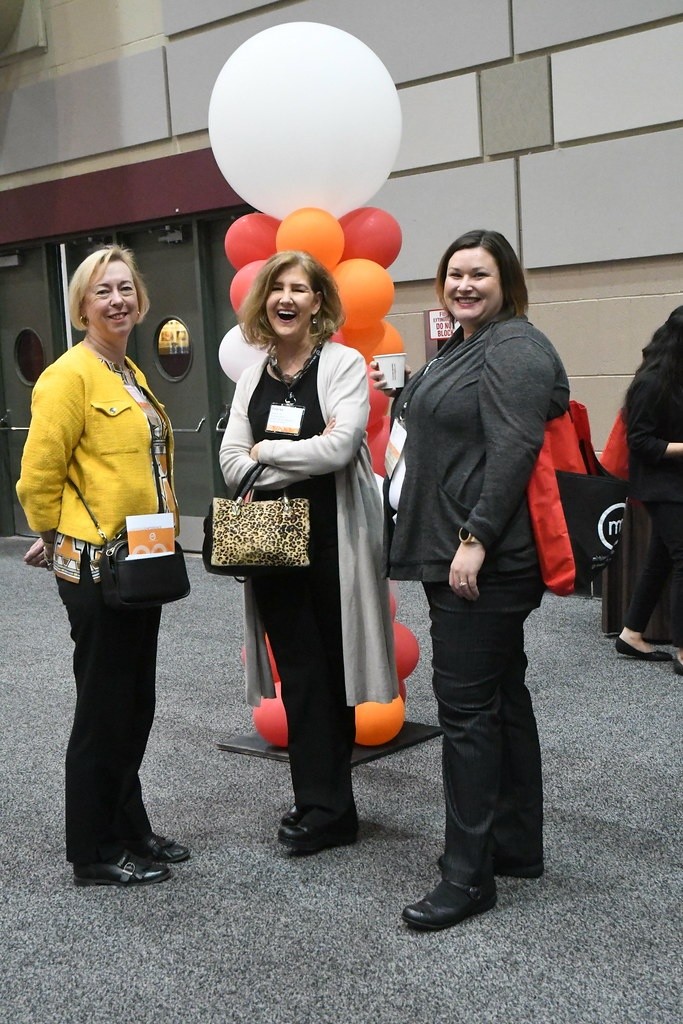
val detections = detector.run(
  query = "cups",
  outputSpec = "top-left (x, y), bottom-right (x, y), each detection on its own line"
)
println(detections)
top-left (373, 352), bottom-right (407, 390)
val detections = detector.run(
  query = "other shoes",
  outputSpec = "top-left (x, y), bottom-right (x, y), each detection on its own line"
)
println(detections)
top-left (616, 637), bottom-right (672, 661)
top-left (674, 654), bottom-right (683, 674)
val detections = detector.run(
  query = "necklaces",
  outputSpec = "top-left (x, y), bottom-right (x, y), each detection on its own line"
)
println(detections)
top-left (268, 340), bottom-right (319, 382)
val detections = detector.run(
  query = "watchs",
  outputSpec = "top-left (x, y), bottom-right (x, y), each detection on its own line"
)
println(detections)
top-left (458, 527), bottom-right (481, 544)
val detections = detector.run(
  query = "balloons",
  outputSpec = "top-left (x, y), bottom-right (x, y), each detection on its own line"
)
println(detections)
top-left (217, 207), bottom-right (419, 746)
top-left (209, 21), bottom-right (402, 221)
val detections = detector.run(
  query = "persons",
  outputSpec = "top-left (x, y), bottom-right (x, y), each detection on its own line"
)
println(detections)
top-left (217, 247), bottom-right (400, 849)
top-left (614, 306), bottom-right (683, 673)
top-left (369, 227), bottom-right (569, 930)
top-left (16, 248), bottom-right (191, 886)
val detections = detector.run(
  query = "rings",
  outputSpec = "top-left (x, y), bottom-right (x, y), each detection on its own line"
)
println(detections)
top-left (458, 582), bottom-right (467, 586)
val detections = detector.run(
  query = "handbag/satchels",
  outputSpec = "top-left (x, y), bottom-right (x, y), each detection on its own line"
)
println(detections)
top-left (483, 337), bottom-right (631, 599)
top-left (100, 525), bottom-right (190, 610)
top-left (203, 465), bottom-right (310, 578)
top-left (599, 403), bottom-right (630, 484)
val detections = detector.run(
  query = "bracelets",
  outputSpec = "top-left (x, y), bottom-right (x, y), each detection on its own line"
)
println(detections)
top-left (42, 546), bottom-right (52, 566)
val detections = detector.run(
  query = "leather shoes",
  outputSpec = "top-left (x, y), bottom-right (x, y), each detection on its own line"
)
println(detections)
top-left (402, 884), bottom-right (498, 930)
top-left (278, 823), bottom-right (356, 849)
top-left (73, 853), bottom-right (171, 886)
top-left (129, 835), bottom-right (190, 862)
top-left (281, 805), bottom-right (302, 826)
top-left (439, 852), bottom-right (544, 878)
